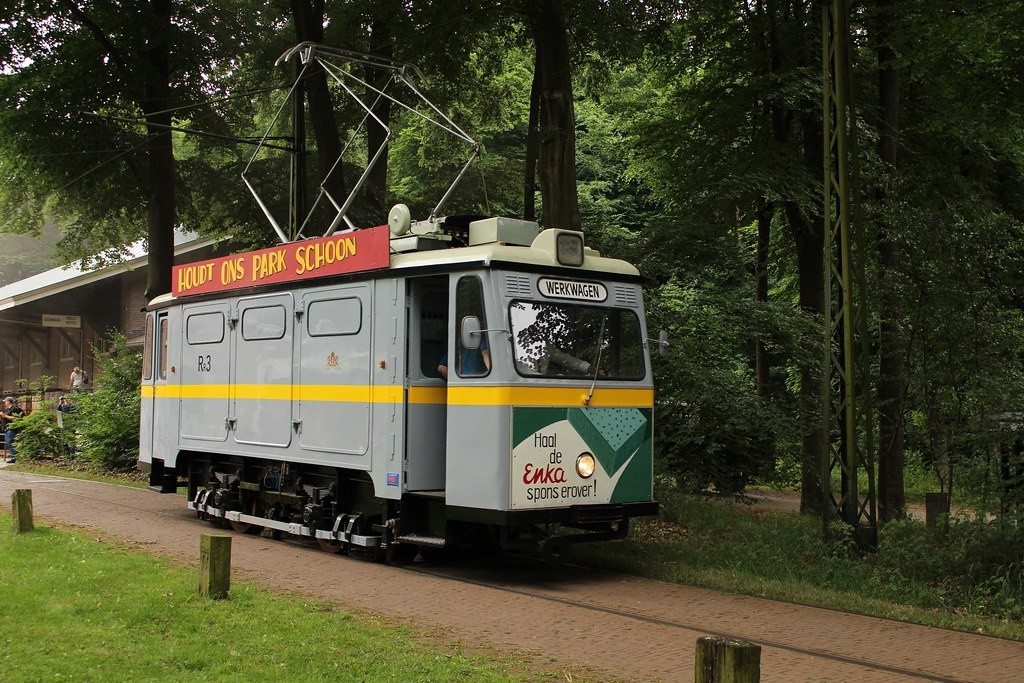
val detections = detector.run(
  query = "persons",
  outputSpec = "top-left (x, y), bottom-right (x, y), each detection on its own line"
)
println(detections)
top-left (57, 367), bottom-right (90, 459)
top-left (0, 396), bottom-right (26, 463)
top-left (437, 320), bottom-right (611, 379)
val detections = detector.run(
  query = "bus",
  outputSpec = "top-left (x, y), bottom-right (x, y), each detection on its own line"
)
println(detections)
top-left (134, 203), bottom-right (671, 567)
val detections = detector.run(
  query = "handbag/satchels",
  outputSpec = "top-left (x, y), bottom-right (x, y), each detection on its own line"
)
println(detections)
top-left (82, 371), bottom-right (88, 383)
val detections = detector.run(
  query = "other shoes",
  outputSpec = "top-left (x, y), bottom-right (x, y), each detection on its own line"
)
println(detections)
top-left (7, 458), bottom-right (17, 463)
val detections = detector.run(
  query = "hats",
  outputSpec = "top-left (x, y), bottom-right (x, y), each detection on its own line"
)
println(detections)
top-left (3, 396), bottom-right (14, 402)
